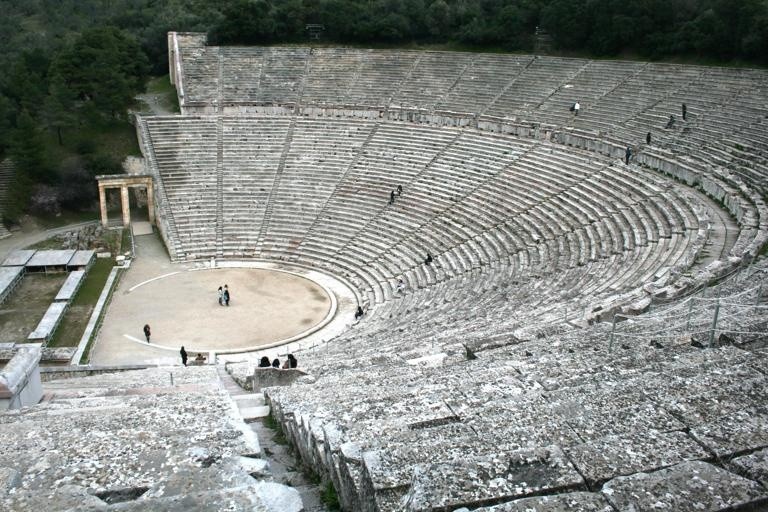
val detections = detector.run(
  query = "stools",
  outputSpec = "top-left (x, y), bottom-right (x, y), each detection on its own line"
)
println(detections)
top-left (141, 103), bottom-right (768, 328)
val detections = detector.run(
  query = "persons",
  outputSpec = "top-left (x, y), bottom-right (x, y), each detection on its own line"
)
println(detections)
top-left (180, 346), bottom-right (188, 367)
top-left (626, 103), bottom-right (687, 165)
top-left (258, 354), bottom-right (297, 369)
top-left (395, 277), bottom-right (406, 292)
top-left (389, 191), bottom-right (395, 204)
top-left (223, 284), bottom-right (230, 306)
top-left (574, 102), bottom-right (580, 116)
top-left (425, 253), bottom-right (432, 265)
top-left (397, 185), bottom-right (402, 196)
top-left (355, 306), bottom-right (364, 318)
top-left (144, 324), bottom-right (151, 343)
top-left (195, 354), bottom-right (206, 360)
top-left (218, 286), bottom-right (224, 306)
